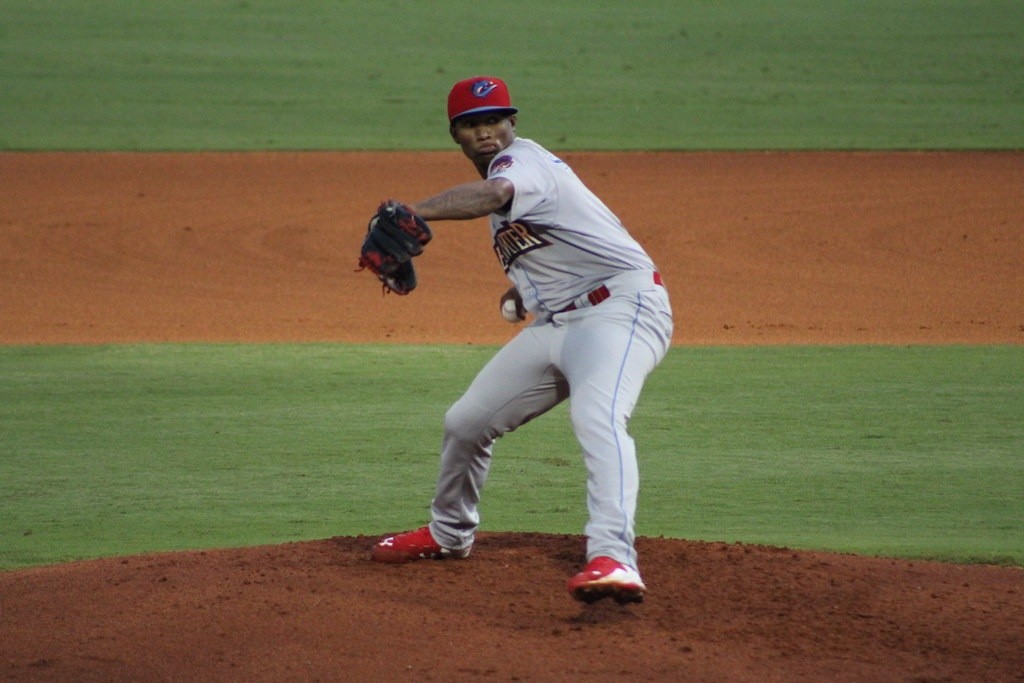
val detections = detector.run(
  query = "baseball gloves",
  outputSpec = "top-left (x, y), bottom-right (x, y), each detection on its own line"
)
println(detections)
top-left (351, 196), bottom-right (433, 297)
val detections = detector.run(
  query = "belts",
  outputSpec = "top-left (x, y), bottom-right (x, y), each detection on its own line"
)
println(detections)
top-left (558, 271), bottom-right (663, 313)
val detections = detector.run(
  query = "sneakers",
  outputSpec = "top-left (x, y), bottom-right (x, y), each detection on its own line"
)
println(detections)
top-left (568, 555), bottom-right (648, 606)
top-left (373, 526), bottom-right (472, 563)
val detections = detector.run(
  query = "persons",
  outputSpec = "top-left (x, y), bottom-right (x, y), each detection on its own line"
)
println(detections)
top-left (371, 77), bottom-right (674, 605)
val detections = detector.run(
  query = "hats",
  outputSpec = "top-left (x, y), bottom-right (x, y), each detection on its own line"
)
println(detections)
top-left (447, 78), bottom-right (518, 123)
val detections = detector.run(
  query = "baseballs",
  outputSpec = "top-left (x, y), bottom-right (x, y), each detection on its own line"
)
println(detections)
top-left (501, 299), bottom-right (525, 323)
